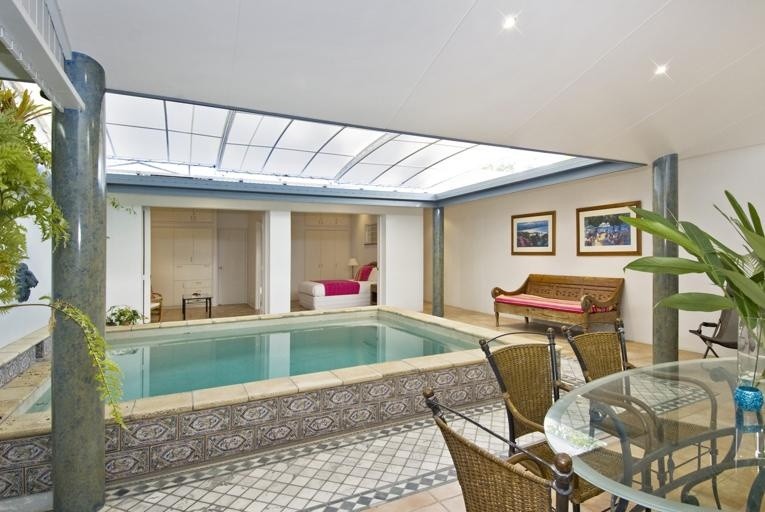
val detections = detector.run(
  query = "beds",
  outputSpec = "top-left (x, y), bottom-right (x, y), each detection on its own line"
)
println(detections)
top-left (299, 264), bottom-right (379, 312)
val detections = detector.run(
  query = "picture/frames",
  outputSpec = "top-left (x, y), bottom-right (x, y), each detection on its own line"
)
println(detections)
top-left (510, 211), bottom-right (556, 255)
top-left (576, 201), bottom-right (642, 258)
top-left (364, 223), bottom-right (377, 244)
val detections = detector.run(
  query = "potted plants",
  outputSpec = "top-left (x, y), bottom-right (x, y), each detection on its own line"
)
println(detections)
top-left (109, 302), bottom-right (148, 329)
top-left (617, 189), bottom-right (764, 411)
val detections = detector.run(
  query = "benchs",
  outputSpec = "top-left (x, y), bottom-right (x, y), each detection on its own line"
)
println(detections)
top-left (490, 275), bottom-right (625, 336)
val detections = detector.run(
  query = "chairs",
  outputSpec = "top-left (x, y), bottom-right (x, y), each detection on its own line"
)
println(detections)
top-left (687, 295), bottom-right (737, 359)
top-left (152, 290), bottom-right (162, 322)
top-left (422, 384), bottom-right (576, 511)
top-left (480, 326), bottom-right (650, 511)
top-left (558, 321), bottom-right (724, 511)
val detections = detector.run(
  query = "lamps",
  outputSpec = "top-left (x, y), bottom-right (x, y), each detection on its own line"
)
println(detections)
top-left (346, 258), bottom-right (358, 279)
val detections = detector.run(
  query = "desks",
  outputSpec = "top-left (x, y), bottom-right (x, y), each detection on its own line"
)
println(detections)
top-left (181, 293), bottom-right (212, 319)
top-left (544, 354), bottom-right (764, 511)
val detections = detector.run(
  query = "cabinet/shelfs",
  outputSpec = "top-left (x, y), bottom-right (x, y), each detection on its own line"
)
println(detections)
top-left (305, 229), bottom-right (353, 282)
top-left (151, 207), bottom-right (214, 223)
top-left (305, 213), bottom-right (351, 228)
top-left (152, 226), bottom-right (214, 308)
